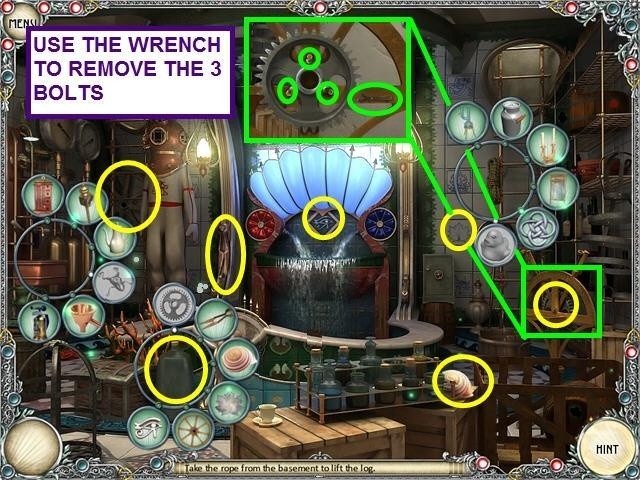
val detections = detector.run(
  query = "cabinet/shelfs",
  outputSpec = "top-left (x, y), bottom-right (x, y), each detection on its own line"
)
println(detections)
top-left (544, 45), bottom-right (631, 334)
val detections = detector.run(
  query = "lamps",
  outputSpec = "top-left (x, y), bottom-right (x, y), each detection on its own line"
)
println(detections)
top-left (185, 119), bottom-right (222, 175)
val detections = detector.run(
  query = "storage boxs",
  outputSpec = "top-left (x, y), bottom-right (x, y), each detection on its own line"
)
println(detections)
top-left (70, 361), bottom-right (150, 421)
top-left (401, 397), bottom-right (476, 458)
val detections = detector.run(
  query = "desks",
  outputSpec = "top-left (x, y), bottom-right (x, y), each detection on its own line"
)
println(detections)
top-left (230, 401), bottom-right (406, 460)
